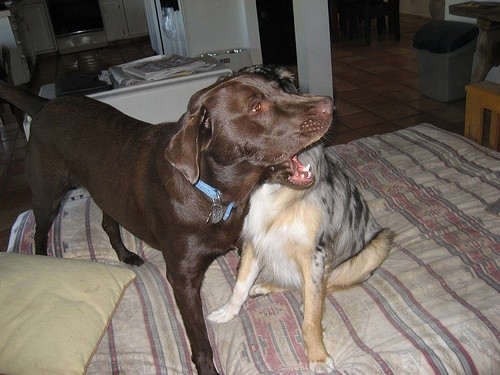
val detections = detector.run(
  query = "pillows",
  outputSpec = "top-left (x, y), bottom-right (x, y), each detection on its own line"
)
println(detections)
top-left (0, 249), bottom-right (136, 375)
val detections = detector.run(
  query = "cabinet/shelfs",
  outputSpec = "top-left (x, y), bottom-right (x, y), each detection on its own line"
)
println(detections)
top-left (14, 0), bottom-right (58, 57)
top-left (55, 27), bottom-right (109, 55)
top-left (99, 0), bottom-right (149, 41)
top-left (0, 4), bottom-right (41, 86)
top-left (22, 54), bottom-right (232, 143)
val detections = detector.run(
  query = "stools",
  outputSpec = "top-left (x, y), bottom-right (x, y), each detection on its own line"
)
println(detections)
top-left (464, 82), bottom-right (500, 150)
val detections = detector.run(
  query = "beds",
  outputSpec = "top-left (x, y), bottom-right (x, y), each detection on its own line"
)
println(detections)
top-left (3, 121), bottom-right (500, 375)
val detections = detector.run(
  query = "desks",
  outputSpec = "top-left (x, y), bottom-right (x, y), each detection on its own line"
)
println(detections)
top-left (328, 0), bottom-right (394, 42)
top-left (448, 1), bottom-right (500, 84)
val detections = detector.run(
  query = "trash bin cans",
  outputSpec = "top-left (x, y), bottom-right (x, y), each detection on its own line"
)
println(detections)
top-left (412, 19), bottom-right (478, 104)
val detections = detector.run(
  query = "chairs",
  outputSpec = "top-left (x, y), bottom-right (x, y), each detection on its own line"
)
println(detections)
top-left (0, 45), bottom-right (17, 117)
top-left (346, 0), bottom-right (401, 44)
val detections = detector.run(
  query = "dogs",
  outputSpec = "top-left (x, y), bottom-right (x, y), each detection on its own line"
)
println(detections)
top-left (0, 64), bottom-right (393, 375)
